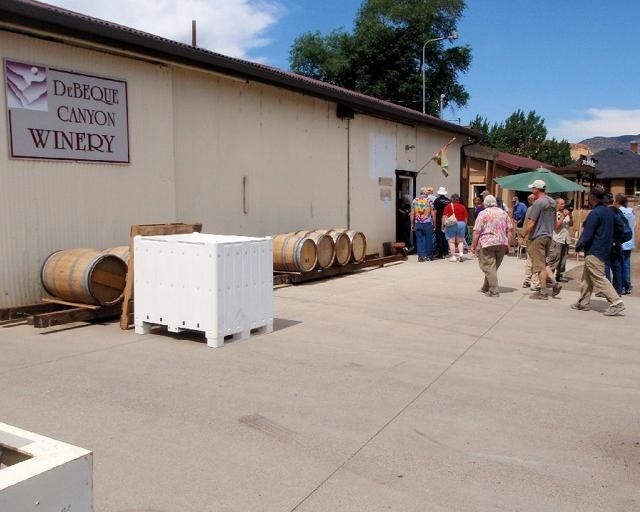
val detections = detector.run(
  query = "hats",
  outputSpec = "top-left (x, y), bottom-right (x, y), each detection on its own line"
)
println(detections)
top-left (480, 190), bottom-right (490, 195)
top-left (528, 180), bottom-right (546, 190)
top-left (512, 196), bottom-right (519, 202)
top-left (420, 187), bottom-right (447, 195)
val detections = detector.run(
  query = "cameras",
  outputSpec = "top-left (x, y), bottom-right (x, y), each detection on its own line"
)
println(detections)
top-left (567, 207), bottom-right (573, 212)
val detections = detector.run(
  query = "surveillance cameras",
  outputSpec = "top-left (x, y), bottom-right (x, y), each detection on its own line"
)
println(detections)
top-left (468, 138), bottom-right (474, 143)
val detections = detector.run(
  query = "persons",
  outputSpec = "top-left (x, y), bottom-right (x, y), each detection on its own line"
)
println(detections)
top-left (604, 193), bottom-right (632, 295)
top-left (571, 186), bottom-right (625, 316)
top-left (614, 193), bottom-right (636, 294)
top-left (470, 195), bottom-right (513, 297)
top-left (518, 180), bottom-right (562, 299)
top-left (402, 188), bottom-right (509, 262)
top-left (523, 195), bottom-right (539, 290)
top-left (546, 197), bottom-right (574, 287)
top-left (512, 195), bottom-right (527, 227)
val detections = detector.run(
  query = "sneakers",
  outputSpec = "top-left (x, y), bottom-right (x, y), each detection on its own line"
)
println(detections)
top-left (522, 276), bottom-right (568, 300)
top-left (480, 287), bottom-right (489, 293)
top-left (569, 301), bottom-right (591, 312)
top-left (483, 290), bottom-right (499, 297)
top-left (603, 301), bottom-right (626, 316)
top-left (418, 253), bottom-right (464, 262)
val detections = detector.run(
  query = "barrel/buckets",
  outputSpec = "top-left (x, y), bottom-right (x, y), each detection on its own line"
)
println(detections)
top-left (272, 227), bottom-right (366, 273)
top-left (38, 246), bottom-right (129, 305)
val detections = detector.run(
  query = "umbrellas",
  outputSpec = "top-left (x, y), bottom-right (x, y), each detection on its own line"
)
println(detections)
top-left (493, 164), bottom-right (585, 193)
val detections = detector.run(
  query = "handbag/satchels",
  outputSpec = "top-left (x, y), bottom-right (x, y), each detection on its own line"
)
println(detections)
top-left (445, 202), bottom-right (457, 227)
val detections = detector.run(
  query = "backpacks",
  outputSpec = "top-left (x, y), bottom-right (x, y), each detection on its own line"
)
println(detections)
top-left (613, 207), bottom-right (631, 243)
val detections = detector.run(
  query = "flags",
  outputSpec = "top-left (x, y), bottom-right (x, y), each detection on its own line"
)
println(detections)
top-left (433, 144), bottom-right (450, 177)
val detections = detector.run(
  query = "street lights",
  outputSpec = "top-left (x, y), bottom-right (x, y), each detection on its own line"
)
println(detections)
top-left (422, 32), bottom-right (458, 114)
top-left (439, 93), bottom-right (447, 119)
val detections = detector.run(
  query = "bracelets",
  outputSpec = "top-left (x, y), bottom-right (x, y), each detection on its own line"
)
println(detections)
top-left (520, 234), bottom-right (525, 240)
